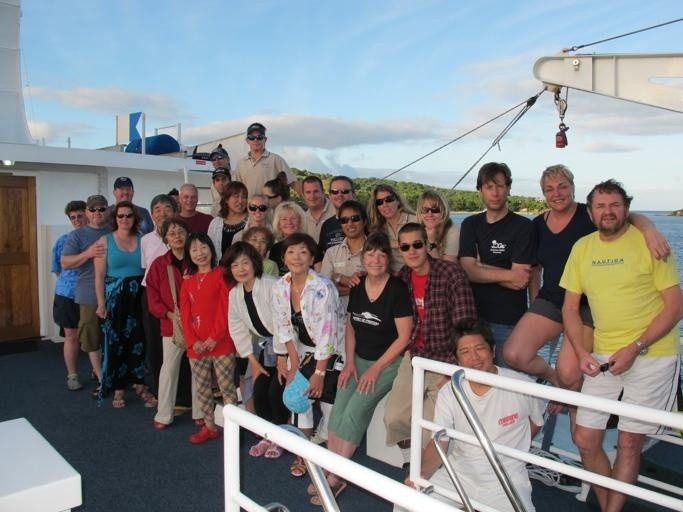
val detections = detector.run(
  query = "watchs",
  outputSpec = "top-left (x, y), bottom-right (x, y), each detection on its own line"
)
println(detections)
top-left (313, 369), bottom-right (327, 377)
top-left (634, 338), bottom-right (648, 355)
top-left (335, 274), bottom-right (341, 284)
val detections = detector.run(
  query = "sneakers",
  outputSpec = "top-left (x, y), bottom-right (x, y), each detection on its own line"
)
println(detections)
top-left (66, 373), bottom-right (83, 390)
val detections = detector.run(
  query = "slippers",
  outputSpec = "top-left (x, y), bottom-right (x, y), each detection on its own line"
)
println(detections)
top-left (307, 474), bottom-right (347, 505)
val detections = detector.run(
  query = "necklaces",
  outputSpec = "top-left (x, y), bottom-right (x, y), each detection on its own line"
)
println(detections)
top-left (370, 277), bottom-right (386, 303)
top-left (293, 281), bottom-right (304, 295)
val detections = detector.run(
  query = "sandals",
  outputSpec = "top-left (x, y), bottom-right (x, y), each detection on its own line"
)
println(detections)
top-left (290, 456), bottom-right (307, 476)
top-left (92, 381), bottom-right (101, 397)
top-left (189, 425), bottom-right (219, 444)
top-left (135, 387), bottom-right (155, 402)
top-left (112, 392), bottom-right (126, 409)
top-left (249, 438), bottom-right (271, 456)
top-left (264, 443), bottom-right (283, 458)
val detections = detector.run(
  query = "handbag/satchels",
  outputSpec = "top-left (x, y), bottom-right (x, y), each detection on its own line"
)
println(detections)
top-left (298, 351), bottom-right (345, 405)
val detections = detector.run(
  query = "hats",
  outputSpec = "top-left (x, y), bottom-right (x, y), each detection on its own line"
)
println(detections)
top-left (211, 167), bottom-right (230, 178)
top-left (112, 176), bottom-right (133, 188)
top-left (87, 194), bottom-right (107, 205)
top-left (210, 148), bottom-right (228, 156)
top-left (246, 122), bottom-right (266, 133)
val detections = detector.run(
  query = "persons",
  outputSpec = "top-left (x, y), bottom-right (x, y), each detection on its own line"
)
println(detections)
top-left (271, 232), bottom-right (347, 476)
top-left (168, 187), bottom-right (181, 213)
top-left (416, 190), bottom-right (459, 264)
top-left (301, 175), bottom-right (336, 245)
top-left (140, 193), bottom-right (192, 417)
top-left (502, 164), bottom-right (671, 444)
top-left (50, 200), bottom-right (89, 391)
top-left (458, 162), bottom-right (538, 372)
top-left (180, 232), bottom-right (238, 445)
top-left (320, 200), bottom-right (367, 310)
top-left (234, 225), bottom-right (279, 403)
top-left (392, 317), bottom-right (546, 512)
top-left (60, 194), bottom-right (116, 398)
top-left (314, 175), bottom-right (371, 255)
top-left (222, 241), bottom-right (290, 459)
top-left (268, 200), bottom-right (323, 279)
top-left (107, 176), bottom-right (155, 235)
top-left (234, 121), bottom-right (302, 202)
top-left (232, 194), bottom-right (282, 245)
top-left (93, 201), bottom-right (158, 410)
top-left (384, 222), bottom-right (479, 478)
top-left (557, 180), bottom-right (683, 512)
top-left (307, 231), bottom-right (415, 505)
top-left (367, 182), bottom-right (418, 276)
top-left (206, 180), bottom-right (250, 266)
top-left (209, 144), bottom-right (236, 202)
top-left (176, 184), bottom-right (214, 233)
top-left (145, 218), bottom-right (205, 430)
top-left (262, 171), bottom-right (291, 227)
top-left (211, 166), bottom-right (232, 218)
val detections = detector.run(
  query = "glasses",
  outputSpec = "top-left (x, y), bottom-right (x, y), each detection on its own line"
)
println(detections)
top-left (400, 243), bottom-right (423, 251)
top-left (421, 207), bottom-right (440, 214)
top-left (247, 134), bottom-right (264, 141)
top-left (116, 213), bottom-right (134, 218)
top-left (375, 194), bottom-right (395, 206)
top-left (340, 215), bottom-right (360, 224)
top-left (88, 207), bottom-right (107, 212)
top-left (211, 156), bottom-right (223, 161)
top-left (331, 189), bottom-right (350, 195)
top-left (267, 194), bottom-right (279, 200)
top-left (249, 204), bottom-right (268, 212)
